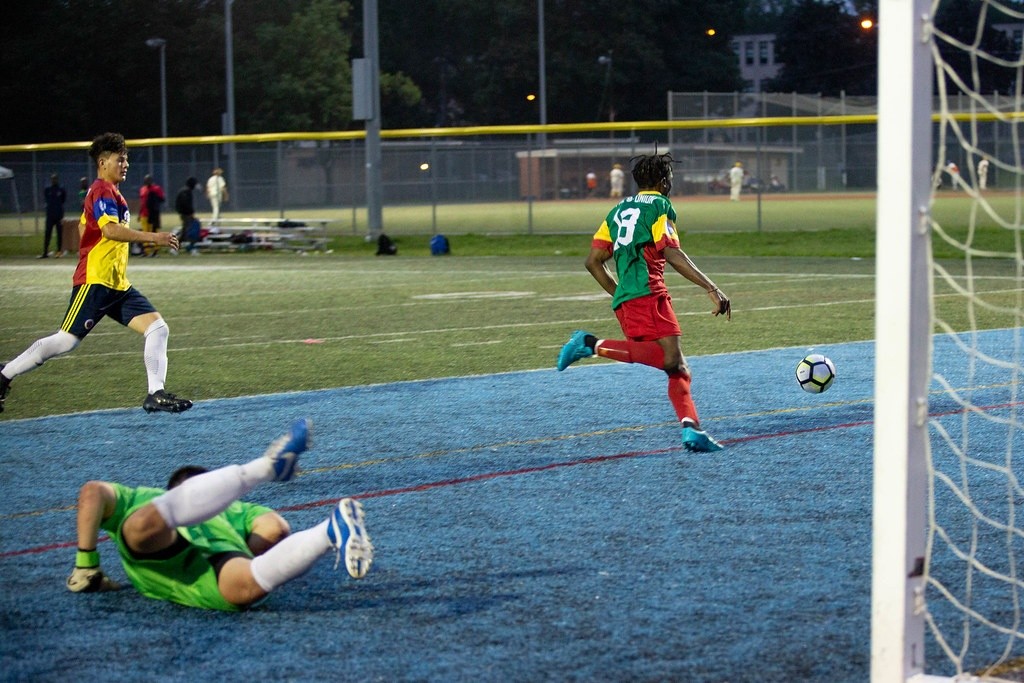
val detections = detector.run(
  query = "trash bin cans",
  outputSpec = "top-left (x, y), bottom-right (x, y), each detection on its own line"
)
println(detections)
top-left (61, 217), bottom-right (81, 250)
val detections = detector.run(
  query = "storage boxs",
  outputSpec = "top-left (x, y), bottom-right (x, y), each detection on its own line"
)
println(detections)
top-left (62, 219), bottom-right (80, 252)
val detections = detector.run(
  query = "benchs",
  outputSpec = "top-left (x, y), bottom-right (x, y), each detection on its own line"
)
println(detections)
top-left (170, 218), bottom-right (340, 252)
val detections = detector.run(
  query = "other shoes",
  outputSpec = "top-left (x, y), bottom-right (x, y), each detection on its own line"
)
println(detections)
top-left (38, 253), bottom-right (48, 259)
top-left (53, 252), bottom-right (63, 258)
top-left (151, 249), bottom-right (160, 258)
top-left (169, 248), bottom-right (178, 256)
top-left (189, 249), bottom-right (201, 256)
top-left (138, 254), bottom-right (147, 258)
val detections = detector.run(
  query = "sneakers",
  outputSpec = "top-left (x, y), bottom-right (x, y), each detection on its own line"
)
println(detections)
top-left (557, 329), bottom-right (595, 371)
top-left (0, 363), bottom-right (12, 413)
top-left (143, 390), bottom-right (193, 413)
top-left (267, 418), bottom-right (310, 483)
top-left (327, 496), bottom-right (373, 579)
top-left (681, 427), bottom-right (724, 452)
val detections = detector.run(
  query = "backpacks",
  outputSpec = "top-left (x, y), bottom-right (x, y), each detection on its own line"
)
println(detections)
top-left (431, 235), bottom-right (450, 257)
top-left (375, 234), bottom-right (397, 256)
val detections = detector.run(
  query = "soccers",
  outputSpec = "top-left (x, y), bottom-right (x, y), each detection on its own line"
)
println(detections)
top-left (794, 353), bottom-right (837, 392)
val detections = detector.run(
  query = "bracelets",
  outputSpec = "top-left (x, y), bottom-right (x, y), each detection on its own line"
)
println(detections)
top-left (708, 288), bottom-right (717, 293)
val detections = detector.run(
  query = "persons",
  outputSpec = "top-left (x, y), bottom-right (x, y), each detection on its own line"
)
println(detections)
top-left (170, 176), bottom-right (202, 256)
top-left (730, 161), bottom-right (743, 201)
top-left (64, 419), bottom-right (375, 616)
top-left (78, 177), bottom-right (90, 210)
top-left (945, 160), bottom-right (960, 190)
top-left (205, 168), bottom-right (230, 225)
top-left (0, 132), bottom-right (194, 415)
top-left (557, 141), bottom-right (733, 453)
top-left (977, 157), bottom-right (989, 189)
top-left (36, 174), bottom-right (65, 260)
top-left (609, 162), bottom-right (624, 201)
top-left (135, 174), bottom-right (165, 258)
